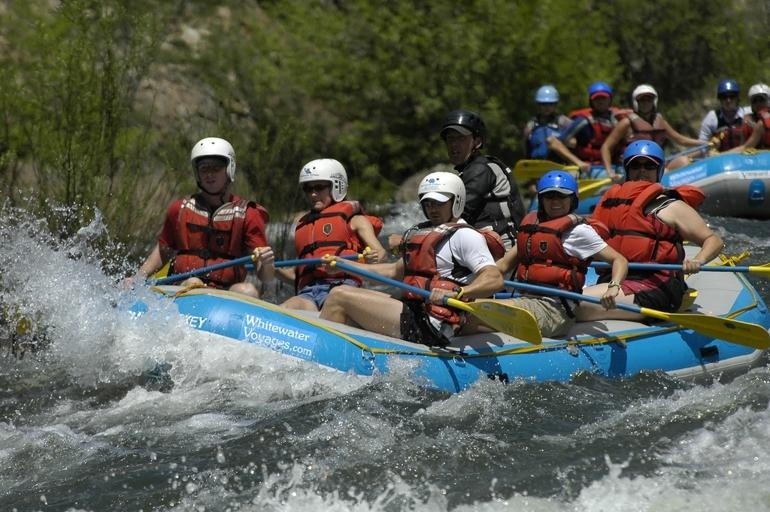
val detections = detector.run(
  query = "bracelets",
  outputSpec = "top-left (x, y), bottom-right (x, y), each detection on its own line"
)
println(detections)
top-left (452, 285), bottom-right (464, 299)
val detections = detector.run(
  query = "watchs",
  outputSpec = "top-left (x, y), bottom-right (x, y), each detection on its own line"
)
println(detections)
top-left (606, 279), bottom-right (621, 290)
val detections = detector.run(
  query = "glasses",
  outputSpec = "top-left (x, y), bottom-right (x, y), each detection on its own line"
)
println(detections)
top-left (301, 183), bottom-right (329, 194)
top-left (626, 160), bottom-right (659, 171)
top-left (720, 95), bottom-right (737, 100)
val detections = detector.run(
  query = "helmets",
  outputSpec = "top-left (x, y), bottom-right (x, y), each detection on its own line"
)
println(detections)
top-left (441, 110), bottom-right (485, 136)
top-left (417, 171), bottom-right (466, 220)
top-left (623, 140), bottom-right (666, 182)
top-left (536, 171), bottom-right (578, 211)
top-left (588, 83), bottom-right (613, 100)
top-left (632, 85), bottom-right (659, 114)
top-left (190, 137), bottom-right (236, 183)
top-left (748, 84), bottom-right (770, 100)
top-left (298, 158), bottom-right (349, 203)
top-left (718, 79), bottom-right (739, 94)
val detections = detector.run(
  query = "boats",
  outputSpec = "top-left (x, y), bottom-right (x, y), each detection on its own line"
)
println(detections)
top-left (113, 247), bottom-right (769, 405)
top-left (525, 146), bottom-right (769, 224)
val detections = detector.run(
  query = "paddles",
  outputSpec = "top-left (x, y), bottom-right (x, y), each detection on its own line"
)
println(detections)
top-left (323, 252), bottom-right (542, 346)
top-left (576, 137), bottom-right (715, 205)
top-left (502, 279), bottom-right (770, 351)
top-left (5, 252), bottom-right (257, 336)
top-left (515, 159), bottom-right (614, 186)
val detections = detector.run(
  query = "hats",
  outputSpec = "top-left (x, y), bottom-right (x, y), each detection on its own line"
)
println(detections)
top-left (636, 93), bottom-right (654, 100)
top-left (591, 92), bottom-right (610, 100)
top-left (536, 85), bottom-right (559, 104)
top-left (439, 125), bottom-right (473, 138)
top-left (538, 188), bottom-right (574, 196)
top-left (626, 155), bottom-right (660, 169)
top-left (419, 192), bottom-right (454, 204)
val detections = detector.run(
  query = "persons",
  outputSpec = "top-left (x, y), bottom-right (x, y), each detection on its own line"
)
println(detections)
top-left (112, 132), bottom-right (276, 303)
top-left (317, 169), bottom-right (505, 345)
top-left (464, 168), bottom-right (629, 336)
top-left (574, 133), bottom-right (724, 319)
top-left (276, 154), bottom-right (391, 316)
top-left (518, 77), bottom-right (770, 179)
top-left (385, 106), bottom-right (528, 250)
